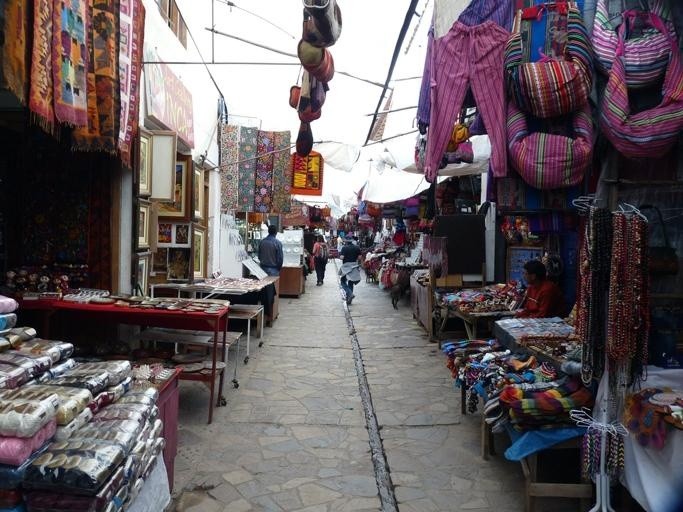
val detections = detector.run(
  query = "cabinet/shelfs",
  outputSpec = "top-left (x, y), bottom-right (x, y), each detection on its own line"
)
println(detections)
top-left (153, 365), bottom-right (184, 495)
top-left (263, 275), bottom-right (281, 327)
top-left (279, 264), bottom-right (305, 299)
top-left (505, 246), bottom-right (544, 288)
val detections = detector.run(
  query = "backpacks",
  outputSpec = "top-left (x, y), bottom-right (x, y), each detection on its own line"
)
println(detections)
top-left (318, 243), bottom-right (328, 261)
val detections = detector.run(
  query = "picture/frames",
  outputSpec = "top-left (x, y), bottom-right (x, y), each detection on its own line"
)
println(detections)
top-left (140, 128), bottom-right (205, 297)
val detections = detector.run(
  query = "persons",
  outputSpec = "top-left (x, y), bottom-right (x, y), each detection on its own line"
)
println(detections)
top-left (339, 237), bottom-right (362, 304)
top-left (311, 234), bottom-right (360, 285)
top-left (258, 225), bottom-right (283, 275)
top-left (515, 260), bottom-right (566, 318)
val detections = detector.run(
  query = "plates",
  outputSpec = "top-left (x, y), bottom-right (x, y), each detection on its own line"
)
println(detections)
top-left (92, 296), bottom-right (116, 304)
top-left (156, 300), bottom-right (225, 314)
top-left (276, 234), bottom-right (302, 253)
top-left (133, 349), bottom-right (225, 373)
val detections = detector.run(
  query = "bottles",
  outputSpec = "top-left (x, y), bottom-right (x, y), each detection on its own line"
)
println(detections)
top-left (499, 215), bottom-right (544, 247)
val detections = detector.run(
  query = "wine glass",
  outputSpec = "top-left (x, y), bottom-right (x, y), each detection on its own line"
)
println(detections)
top-left (387, 230), bottom-right (395, 246)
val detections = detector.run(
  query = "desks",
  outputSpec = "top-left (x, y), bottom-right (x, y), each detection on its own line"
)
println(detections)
top-left (409, 269), bottom-right (683, 512)
top-left (9, 290), bottom-right (230, 425)
top-left (133, 303), bottom-right (265, 407)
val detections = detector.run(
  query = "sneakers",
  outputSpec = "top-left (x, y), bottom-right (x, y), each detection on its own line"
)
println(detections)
top-left (346, 293), bottom-right (355, 306)
